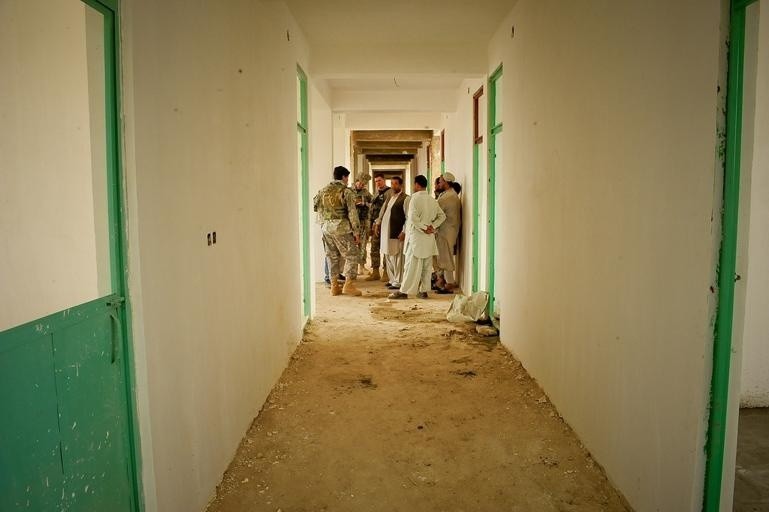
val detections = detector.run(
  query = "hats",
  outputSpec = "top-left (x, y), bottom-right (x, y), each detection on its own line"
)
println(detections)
top-left (356, 171), bottom-right (371, 184)
top-left (443, 172), bottom-right (455, 183)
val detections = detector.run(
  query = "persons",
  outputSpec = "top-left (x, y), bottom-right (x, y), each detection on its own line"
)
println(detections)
top-left (311, 164), bottom-right (361, 297)
top-left (324, 247), bottom-right (346, 284)
top-left (351, 171), bottom-right (462, 300)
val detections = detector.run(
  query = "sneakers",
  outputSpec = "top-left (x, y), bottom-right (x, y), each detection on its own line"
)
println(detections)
top-left (381, 270), bottom-right (454, 299)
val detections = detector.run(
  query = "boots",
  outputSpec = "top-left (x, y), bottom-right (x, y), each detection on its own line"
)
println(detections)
top-left (330, 279), bottom-right (342, 295)
top-left (342, 281), bottom-right (361, 295)
top-left (325, 265), bottom-right (380, 283)
